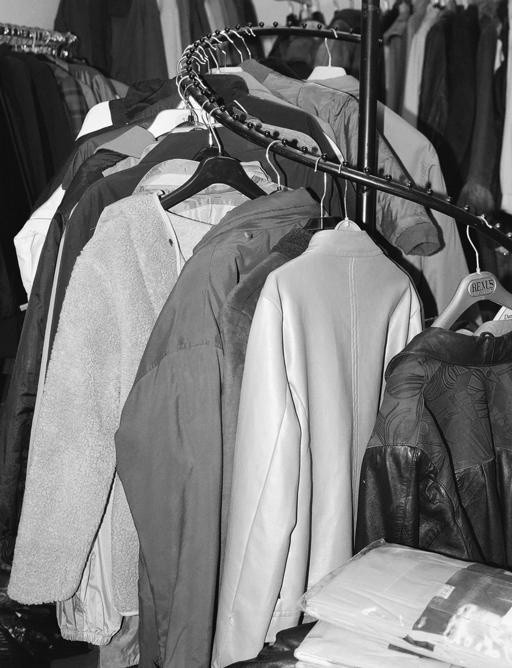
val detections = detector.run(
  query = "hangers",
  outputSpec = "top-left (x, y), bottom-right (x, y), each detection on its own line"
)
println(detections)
top-left (384, 215), bottom-right (511, 407)
